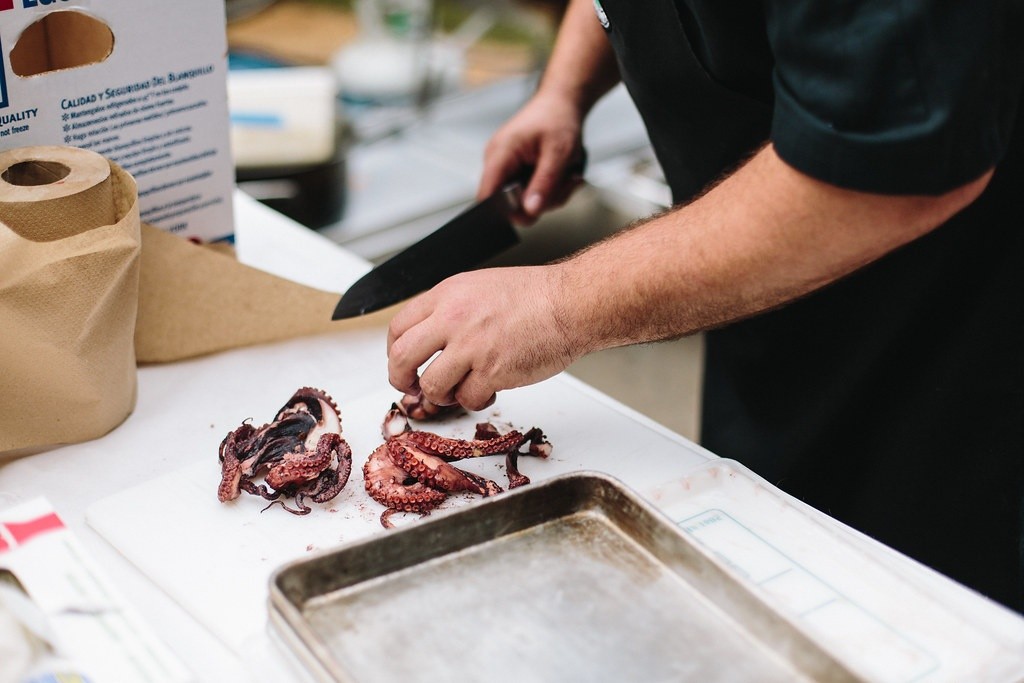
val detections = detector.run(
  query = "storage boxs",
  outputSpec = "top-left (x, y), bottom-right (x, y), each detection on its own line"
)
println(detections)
top-left (228, 63), bottom-right (352, 231)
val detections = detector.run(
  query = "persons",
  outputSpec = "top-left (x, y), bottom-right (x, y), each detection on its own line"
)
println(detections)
top-left (387, 0), bottom-right (1024, 618)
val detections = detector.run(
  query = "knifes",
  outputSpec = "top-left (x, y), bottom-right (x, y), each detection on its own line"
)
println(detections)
top-left (332, 145), bottom-right (588, 321)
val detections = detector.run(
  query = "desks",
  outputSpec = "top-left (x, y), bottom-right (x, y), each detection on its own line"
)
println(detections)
top-left (0, 190), bottom-right (1024, 683)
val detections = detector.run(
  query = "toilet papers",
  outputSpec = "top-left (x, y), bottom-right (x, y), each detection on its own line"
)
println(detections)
top-left (0, 144), bottom-right (427, 464)
